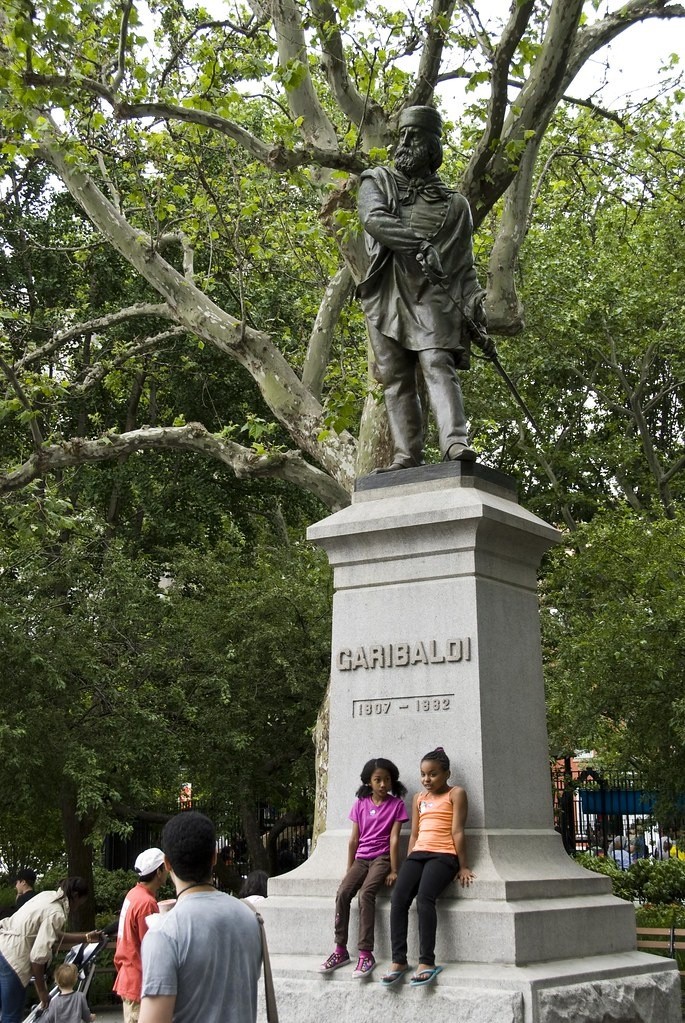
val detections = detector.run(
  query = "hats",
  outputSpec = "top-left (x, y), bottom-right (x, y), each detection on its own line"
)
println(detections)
top-left (134, 847), bottom-right (165, 877)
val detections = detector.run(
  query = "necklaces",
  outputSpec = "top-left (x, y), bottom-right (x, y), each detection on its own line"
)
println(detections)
top-left (175, 882), bottom-right (215, 899)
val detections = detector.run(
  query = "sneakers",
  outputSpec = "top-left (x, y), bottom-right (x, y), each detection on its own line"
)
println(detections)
top-left (352, 954), bottom-right (376, 978)
top-left (316, 951), bottom-right (351, 973)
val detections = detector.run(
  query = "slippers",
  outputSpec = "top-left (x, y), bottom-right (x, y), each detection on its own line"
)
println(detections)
top-left (410, 966), bottom-right (443, 985)
top-left (381, 965), bottom-right (412, 987)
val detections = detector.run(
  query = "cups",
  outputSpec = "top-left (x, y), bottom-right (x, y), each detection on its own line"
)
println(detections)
top-left (157, 899), bottom-right (176, 914)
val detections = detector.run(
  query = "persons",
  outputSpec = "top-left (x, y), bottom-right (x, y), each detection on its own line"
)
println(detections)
top-left (358, 106), bottom-right (497, 474)
top-left (317, 758), bottom-right (409, 978)
top-left (0, 813), bottom-right (313, 1023)
top-left (381, 747), bottom-right (477, 986)
top-left (588, 817), bottom-right (685, 906)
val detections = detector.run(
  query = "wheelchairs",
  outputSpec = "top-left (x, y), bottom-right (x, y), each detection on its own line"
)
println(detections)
top-left (22, 931), bottom-right (110, 1023)
